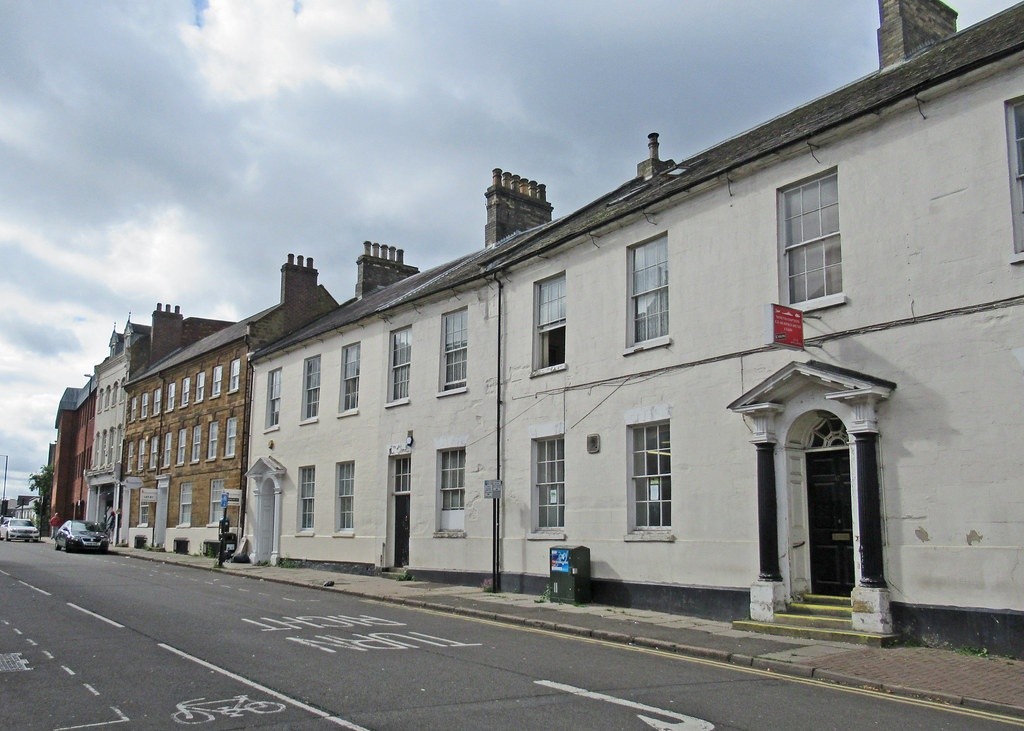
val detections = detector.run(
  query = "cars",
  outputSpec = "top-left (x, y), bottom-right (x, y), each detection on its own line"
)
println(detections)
top-left (54, 520), bottom-right (109, 554)
top-left (0, 519), bottom-right (39, 542)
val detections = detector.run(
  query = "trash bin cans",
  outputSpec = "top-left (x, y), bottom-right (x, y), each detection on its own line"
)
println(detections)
top-left (550, 545), bottom-right (591, 604)
top-left (219, 533), bottom-right (237, 558)
